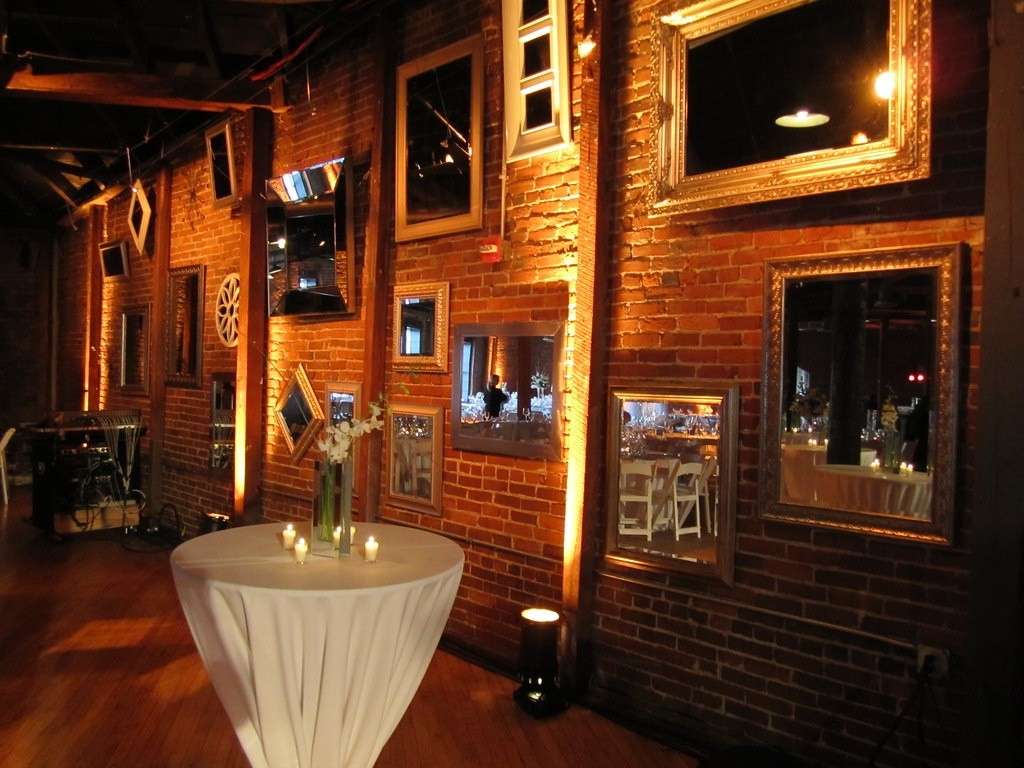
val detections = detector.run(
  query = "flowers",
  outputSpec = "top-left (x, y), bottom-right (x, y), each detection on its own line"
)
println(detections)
top-left (881, 398), bottom-right (898, 431)
top-left (234, 327), bottom-right (425, 543)
top-left (788, 388), bottom-right (826, 418)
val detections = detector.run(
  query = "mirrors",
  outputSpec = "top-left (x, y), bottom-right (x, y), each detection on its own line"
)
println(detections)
top-left (98, 239), bottom-right (132, 284)
top-left (127, 178), bottom-right (152, 256)
top-left (164, 264), bottom-right (204, 389)
top-left (598, 385), bottom-right (741, 589)
top-left (450, 322), bottom-right (568, 462)
top-left (120, 302), bottom-right (152, 397)
top-left (651, 0), bottom-right (933, 219)
top-left (209, 371), bottom-right (236, 480)
top-left (757, 240), bottom-right (963, 545)
top-left (273, 362), bottom-right (325, 466)
top-left (396, 32), bottom-right (485, 243)
top-left (325, 381), bottom-right (363, 498)
top-left (392, 280), bottom-right (450, 375)
top-left (264, 158), bottom-right (356, 318)
top-left (205, 117), bottom-right (239, 210)
top-left (385, 405), bottom-right (445, 517)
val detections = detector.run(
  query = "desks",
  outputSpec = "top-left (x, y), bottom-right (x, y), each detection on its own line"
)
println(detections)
top-left (781, 445), bottom-right (877, 504)
top-left (781, 432), bottom-right (828, 445)
top-left (813, 465), bottom-right (932, 519)
top-left (170, 522), bottom-right (465, 768)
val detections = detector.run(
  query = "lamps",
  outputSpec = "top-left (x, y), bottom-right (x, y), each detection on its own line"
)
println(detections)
top-left (203, 513), bottom-right (231, 534)
top-left (513, 608), bottom-right (570, 721)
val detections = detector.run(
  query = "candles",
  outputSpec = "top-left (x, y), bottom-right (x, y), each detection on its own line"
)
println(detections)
top-left (349, 527), bottom-right (355, 545)
top-left (900, 462), bottom-right (913, 478)
top-left (295, 538), bottom-right (308, 564)
top-left (808, 438), bottom-right (817, 448)
top-left (364, 537), bottom-right (379, 563)
top-left (824, 439), bottom-right (828, 448)
top-left (334, 526), bottom-right (341, 550)
top-left (870, 458), bottom-right (880, 472)
top-left (282, 524), bottom-right (296, 550)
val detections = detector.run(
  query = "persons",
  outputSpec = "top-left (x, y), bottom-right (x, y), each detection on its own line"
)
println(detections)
top-left (483, 375), bottom-right (510, 420)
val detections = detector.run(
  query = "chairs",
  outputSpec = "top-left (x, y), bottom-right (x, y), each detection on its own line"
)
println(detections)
top-left (618, 445), bottom-right (719, 541)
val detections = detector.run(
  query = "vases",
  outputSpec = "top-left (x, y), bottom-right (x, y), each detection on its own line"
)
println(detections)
top-left (883, 429), bottom-right (901, 474)
top-left (311, 461), bottom-right (352, 559)
top-left (800, 416), bottom-right (812, 432)
